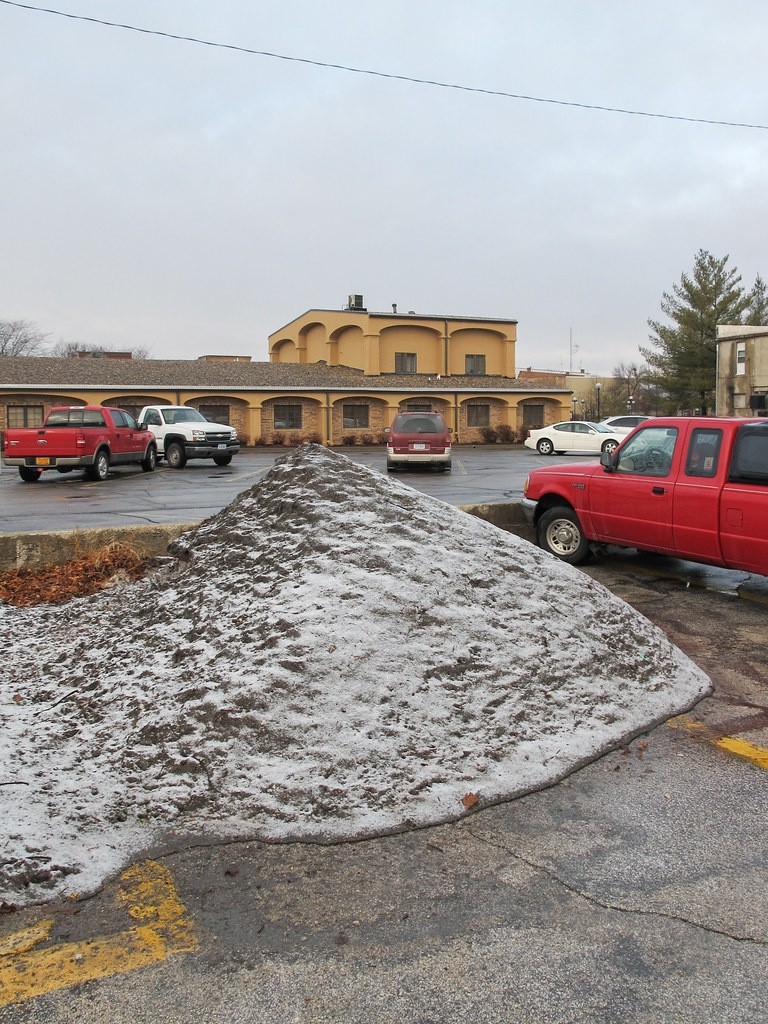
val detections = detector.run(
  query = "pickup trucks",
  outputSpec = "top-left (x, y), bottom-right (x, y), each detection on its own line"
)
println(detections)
top-left (518, 417), bottom-right (768, 579)
top-left (4, 406), bottom-right (158, 480)
top-left (136, 404), bottom-right (241, 469)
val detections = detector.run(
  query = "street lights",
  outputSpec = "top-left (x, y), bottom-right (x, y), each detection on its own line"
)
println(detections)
top-left (627, 396), bottom-right (636, 415)
top-left (595, 382), bottom-right (602, 422)
top-left (572, 397), bottom-right (578, 420)
top-left (580, 398), bottom-right (586, 420)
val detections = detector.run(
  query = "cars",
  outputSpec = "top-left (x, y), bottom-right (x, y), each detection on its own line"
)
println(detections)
top-left (523, 421), bottom-right (647, 457)
top-left (589, 415), bottom-right (674, 449)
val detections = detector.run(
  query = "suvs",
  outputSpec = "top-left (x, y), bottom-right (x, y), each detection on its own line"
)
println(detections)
top-left (383, 410), bottom-right (454, 475)
